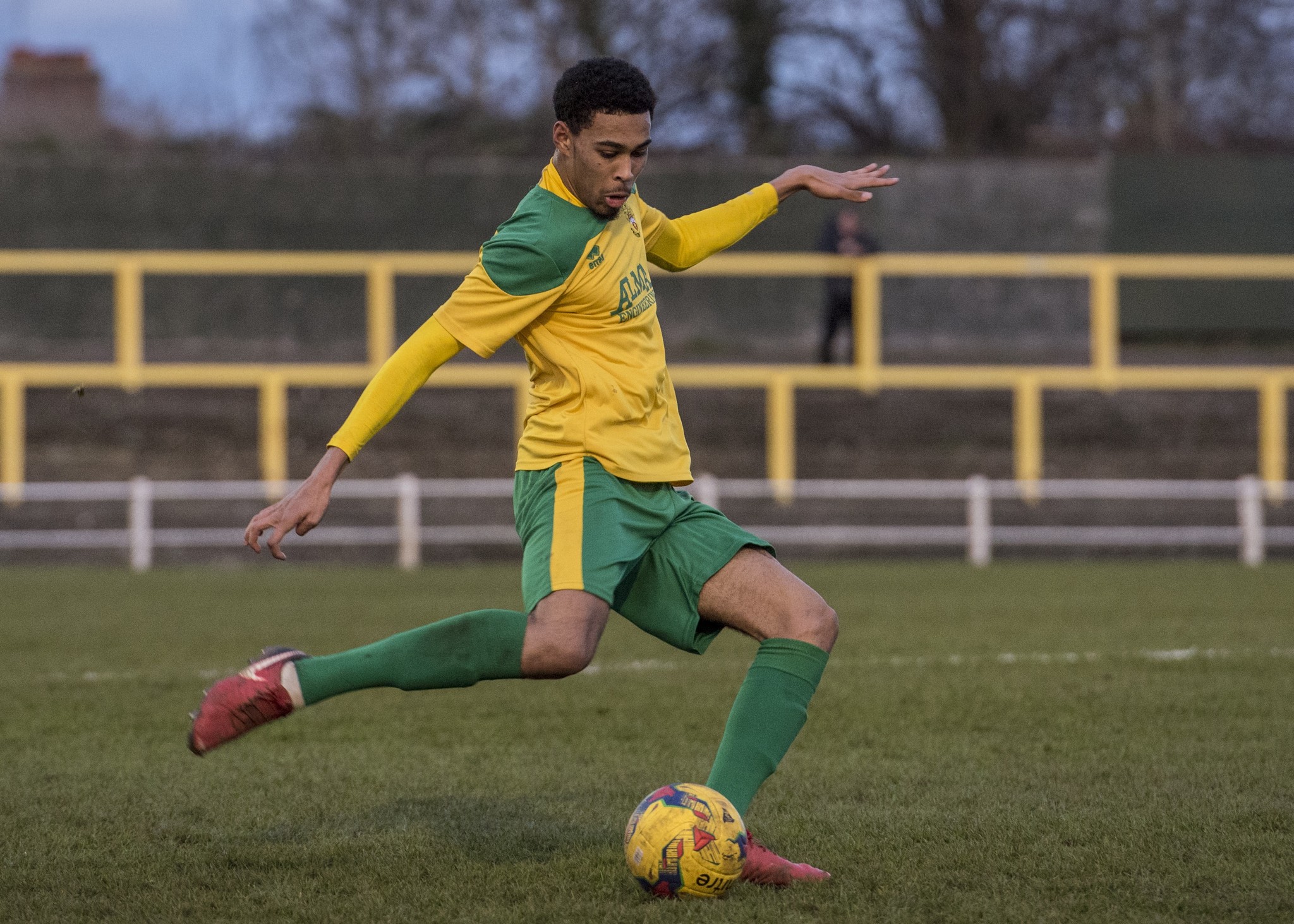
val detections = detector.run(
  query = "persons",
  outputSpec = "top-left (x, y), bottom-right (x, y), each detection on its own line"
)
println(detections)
top-left (185, 56), bottom-right (898, 887)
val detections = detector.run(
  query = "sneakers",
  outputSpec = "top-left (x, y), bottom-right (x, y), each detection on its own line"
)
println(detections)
top-left (741, 828), bottom-right (833, 892)
top-left (187, 647), bottom-right (314, 759)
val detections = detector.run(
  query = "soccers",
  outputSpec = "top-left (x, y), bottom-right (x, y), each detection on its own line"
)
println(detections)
top-left (621, 781), bottom-right (752, 898)
